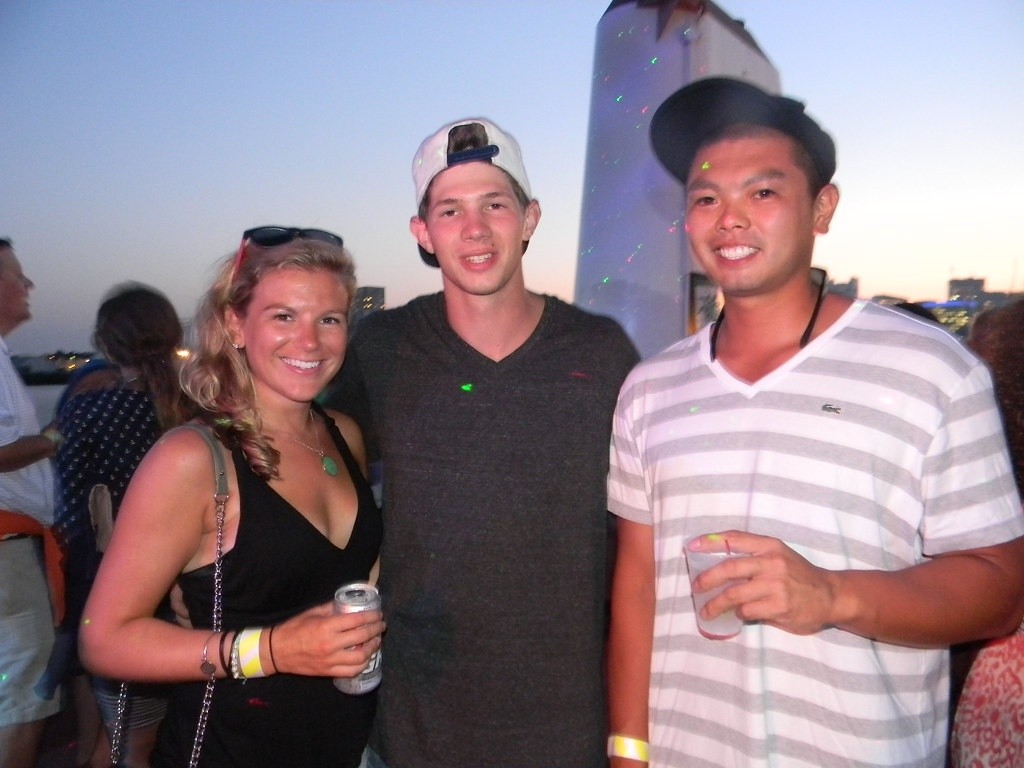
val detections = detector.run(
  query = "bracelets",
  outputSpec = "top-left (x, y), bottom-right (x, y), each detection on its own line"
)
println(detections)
top-left (200, 625), bottom-right (279, 682)
top-left (45, 427), bottom-right (57, 440)
top-left (606, 734), bottom-right (650, 762)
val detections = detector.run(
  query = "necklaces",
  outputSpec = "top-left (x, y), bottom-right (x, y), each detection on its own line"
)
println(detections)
top-left (277, 410), bottom-right (339, 478)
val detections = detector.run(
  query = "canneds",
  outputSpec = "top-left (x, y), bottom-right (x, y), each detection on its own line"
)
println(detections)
top-left (333, 583), bottom-right (382, 694)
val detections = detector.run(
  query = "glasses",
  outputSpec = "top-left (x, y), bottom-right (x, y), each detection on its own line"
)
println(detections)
top-left (230, 226), bottom-right (344, 284)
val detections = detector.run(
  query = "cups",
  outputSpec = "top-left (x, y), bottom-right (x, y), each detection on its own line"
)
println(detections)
top-left (682, 532), bottom-right (746, 637)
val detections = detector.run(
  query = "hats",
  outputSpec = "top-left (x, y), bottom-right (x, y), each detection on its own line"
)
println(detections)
top-left (411, 116), bottom-right (533, 269)
top-left (650, 77), bottom-right (836, 187)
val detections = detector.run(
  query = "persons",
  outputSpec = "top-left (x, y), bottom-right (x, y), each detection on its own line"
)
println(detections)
top-left (605, 73), bottom-right (1024, 768)
top-left (74, 225), bottom-right (382, 768)
top-left (312, 116), bottom-right (644, 768)
top-left (0, 235), bottom-right (202, 768)
